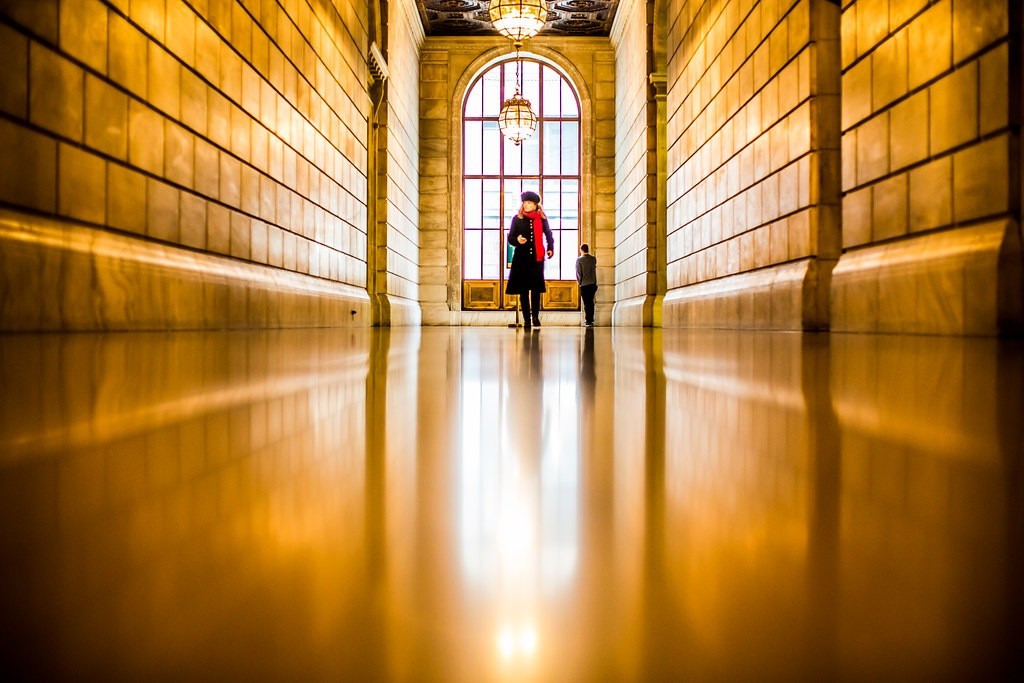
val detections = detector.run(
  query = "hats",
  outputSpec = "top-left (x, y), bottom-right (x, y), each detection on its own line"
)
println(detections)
top-left (520, 191), bottom-right (541, 203)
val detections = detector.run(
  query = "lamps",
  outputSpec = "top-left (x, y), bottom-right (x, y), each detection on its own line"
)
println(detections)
top-left (499, 42), bottom-right (537, 145)
top-left (489, 0), bottom-right (547, 49)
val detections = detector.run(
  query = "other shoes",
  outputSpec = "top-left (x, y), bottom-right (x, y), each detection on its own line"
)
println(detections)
top-left (585, 322), bottom-right (593, 327)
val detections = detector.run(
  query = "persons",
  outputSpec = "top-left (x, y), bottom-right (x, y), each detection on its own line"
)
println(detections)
top-left (505, 192), bottom-right (554, 327)
top-left (575, 243), bottom-right (598, 327)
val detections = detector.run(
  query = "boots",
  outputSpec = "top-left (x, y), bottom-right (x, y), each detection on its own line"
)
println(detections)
top-left (519, 296), bottom-right (532, 329)
top-left (531, 292), bottom-right (541, 326)
